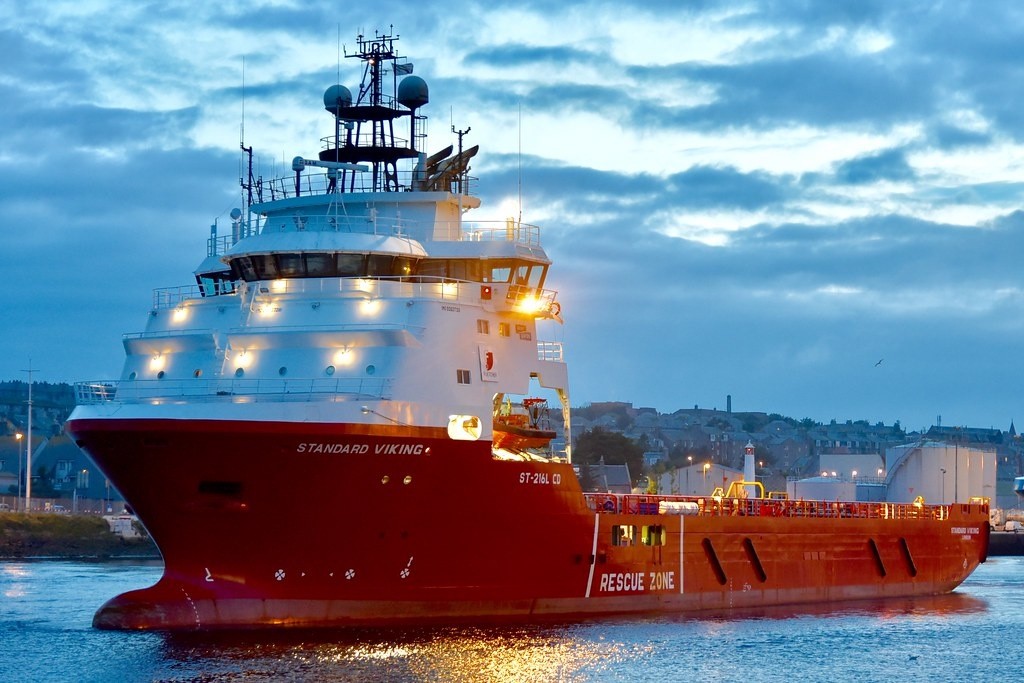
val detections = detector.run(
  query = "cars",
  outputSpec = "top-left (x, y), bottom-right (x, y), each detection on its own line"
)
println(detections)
top-left (53, 504), bottom-right (64, 509)
top-left (0, 504), bottom-right (10, 512)
top-left (1005, 520), bottom-right (1024, 532)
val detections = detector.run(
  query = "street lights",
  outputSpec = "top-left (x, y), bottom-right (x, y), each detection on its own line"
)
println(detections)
top-left (16, 434), bottom-right (22, 512)
top-left (940, 468), bottom-right (946, 502)
top-left (759, 461), bottom-right (763, 484)
top-left (703, 464), bottom-right (710, 486)
top-left (688, 457), bottom-right (692, 494)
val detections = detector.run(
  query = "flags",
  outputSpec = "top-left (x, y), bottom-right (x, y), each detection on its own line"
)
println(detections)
top-left (391, 63), bottom-right (413, 76)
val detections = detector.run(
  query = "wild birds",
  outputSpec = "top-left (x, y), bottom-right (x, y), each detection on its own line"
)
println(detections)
top-left (875, 358), bottom-right (884, 367)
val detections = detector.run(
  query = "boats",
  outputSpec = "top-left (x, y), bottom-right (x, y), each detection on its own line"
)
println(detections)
top-left (64, 23), bottom-right (990, 633)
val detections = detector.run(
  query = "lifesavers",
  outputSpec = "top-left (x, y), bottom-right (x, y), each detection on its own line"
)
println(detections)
top-left (549, 301), bottom-right (561, 316)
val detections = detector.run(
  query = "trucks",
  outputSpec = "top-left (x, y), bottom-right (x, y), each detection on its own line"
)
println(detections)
top-left (989, 509), bottom-right (1024, 531)
